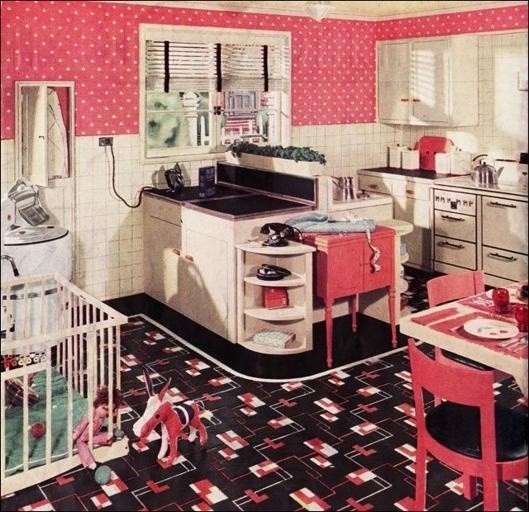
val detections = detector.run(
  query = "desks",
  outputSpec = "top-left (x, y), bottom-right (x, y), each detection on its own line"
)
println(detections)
top-left (295, 226), bottom-right (397, 368)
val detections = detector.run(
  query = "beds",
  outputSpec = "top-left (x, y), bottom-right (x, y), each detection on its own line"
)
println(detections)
top-left (0, 272), bottom-right (129, 497)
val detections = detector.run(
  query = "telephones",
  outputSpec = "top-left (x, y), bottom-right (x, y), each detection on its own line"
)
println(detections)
top-left (260, 223), bottom-right (295, 247)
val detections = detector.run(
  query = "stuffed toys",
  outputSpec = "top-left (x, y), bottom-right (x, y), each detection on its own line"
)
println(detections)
top-left (68, 386), bottom-right (127, 470)
top-left (133, 365), bottom-right (209, 471)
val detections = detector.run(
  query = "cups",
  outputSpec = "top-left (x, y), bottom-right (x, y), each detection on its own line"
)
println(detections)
top-left (492, 288), bottom-right (509, 314)
top-left (516, 306), bottom-right (529, 332)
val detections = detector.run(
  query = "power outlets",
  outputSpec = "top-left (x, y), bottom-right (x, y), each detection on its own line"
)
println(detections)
top-left (99, 137), bottom-right (113, 146)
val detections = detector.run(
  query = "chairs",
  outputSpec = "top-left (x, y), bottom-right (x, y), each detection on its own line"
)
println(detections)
top-left (408, 339), bottom-right (529, 512)
top-left (426, 271), bottom-right (512, 408)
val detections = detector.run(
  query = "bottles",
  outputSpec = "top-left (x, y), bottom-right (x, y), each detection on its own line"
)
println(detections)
top-left (388, 144), bottom-right (419, 170)
top-left (434, 148), bottom-right (472, 176)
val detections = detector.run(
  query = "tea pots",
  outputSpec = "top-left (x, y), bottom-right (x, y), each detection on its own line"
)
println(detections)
top-left (331, 175), bottom-right (357, 202)
top-left (467, 150), bottom-right (504, 187)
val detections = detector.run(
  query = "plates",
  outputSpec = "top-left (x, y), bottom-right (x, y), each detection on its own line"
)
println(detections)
top-left (463, 318), bottom-right (519, 341)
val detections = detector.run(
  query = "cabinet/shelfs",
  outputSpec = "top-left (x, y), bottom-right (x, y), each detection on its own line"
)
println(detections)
top-left (358, 175), bottom-right (432, 272)
top-left (434, 188), bottom-right (528, 287)
top-left (359, 219), bottom-right (411, 325)
top-left (143, 195), bottom-right (311, 345)
top-left (376, 36), bottom-right (479, 126)
top-left (236, 239), bottom-right (313, 356)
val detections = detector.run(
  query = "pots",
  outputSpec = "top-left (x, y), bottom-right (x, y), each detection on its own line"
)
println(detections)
top-left (1, 224), bottom-right (74, 355)
